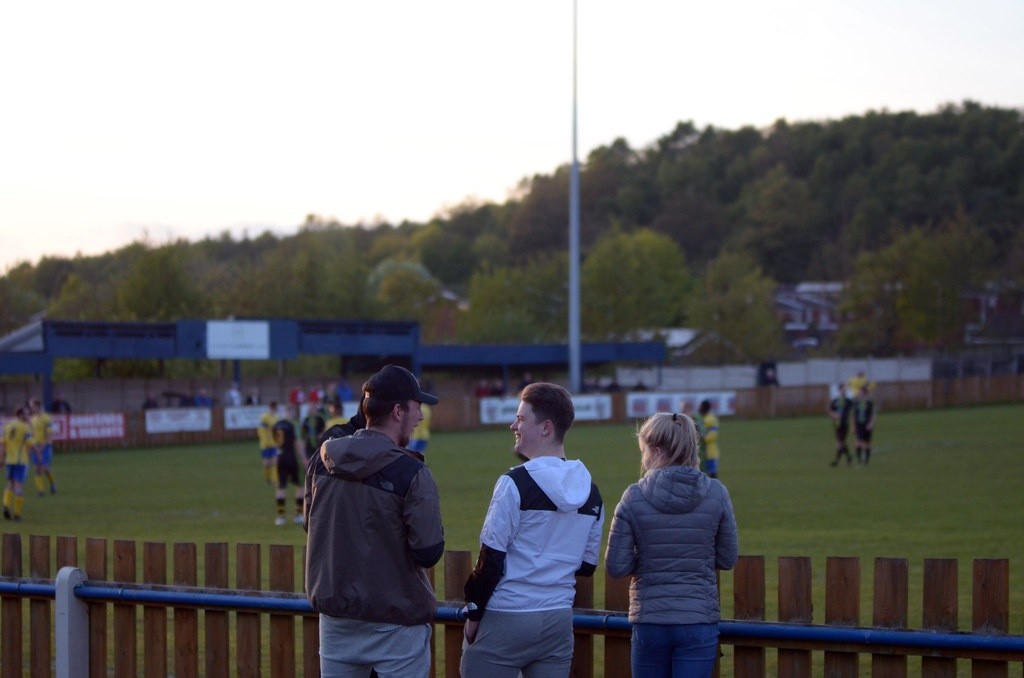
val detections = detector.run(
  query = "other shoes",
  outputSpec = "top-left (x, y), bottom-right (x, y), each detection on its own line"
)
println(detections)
top-left (3, 506), bottom-right (11, 520)
top-left (14, 516), bottom-right (22, 521)
top-left (51, 485), bottom-right (56, 492)
top-left (294, 516), bottom-right (304, 523)
top-left (37, 492), bottom-right (44, 496)
top-left (275, 519), bottom-right (286, 525)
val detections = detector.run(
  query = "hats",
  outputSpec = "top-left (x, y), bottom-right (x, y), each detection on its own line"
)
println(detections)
top-left (364, 364), bottom-right (439, 406)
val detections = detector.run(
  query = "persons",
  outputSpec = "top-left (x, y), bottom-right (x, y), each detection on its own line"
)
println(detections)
top-left (826, 371), bottom-right (876, 468)
top-left (580, 377), bottom-right (649, 393)
top-left (0, 398), bottom-right (56, 521)
top-left (303, 366), bottom-right (445, 678)
top-left (682, 398), bottom-right (719, 479)
top-left (604, 412), bottom-right (738, 678)
top-left (143, 380), bottom-right (350, 526)
top-left (406, 372), bottom-right (534, 455)
top-left (458, 382), bottom-right (604, 678)
